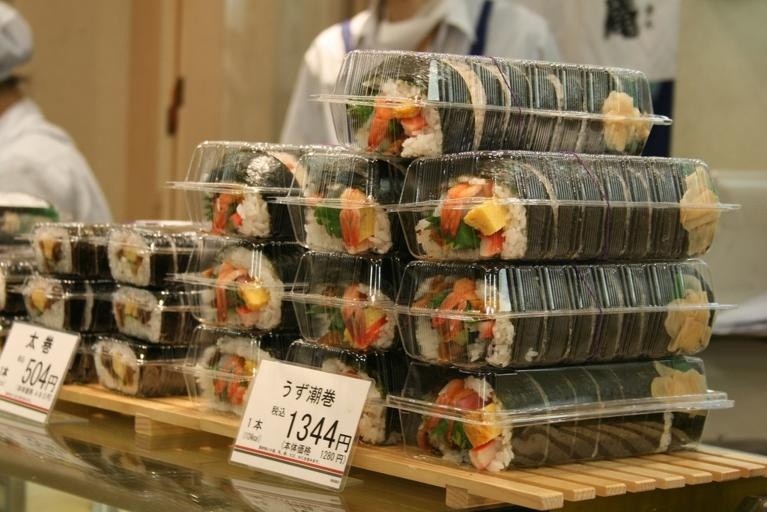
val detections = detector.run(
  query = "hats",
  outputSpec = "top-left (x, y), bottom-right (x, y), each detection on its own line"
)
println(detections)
top-left (0, 1), bottom-right (32, 82)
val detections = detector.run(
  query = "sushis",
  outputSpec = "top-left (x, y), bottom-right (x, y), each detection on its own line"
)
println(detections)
top-left (1, 49), bottom-right (723, 471)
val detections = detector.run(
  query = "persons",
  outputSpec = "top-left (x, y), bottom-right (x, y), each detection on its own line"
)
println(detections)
top-left (579, 0), bottom-right (679, 155)
top-left (0, 0), bottom-right (115, 223)
top-left (278, 0), bottom-right (563, 146)
